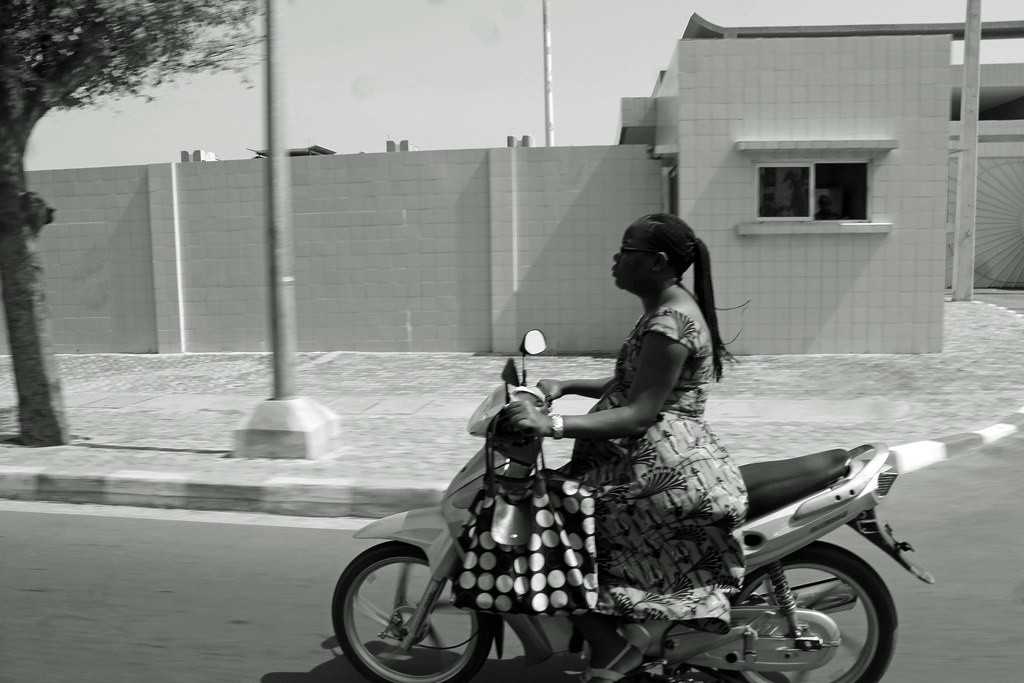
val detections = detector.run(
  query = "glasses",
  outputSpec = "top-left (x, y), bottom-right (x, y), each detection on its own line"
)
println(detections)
top-left (619, 244), bottom-right (657, 257)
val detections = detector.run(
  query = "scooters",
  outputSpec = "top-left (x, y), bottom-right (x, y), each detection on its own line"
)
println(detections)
top-left (329, 327), bottom-right (938, 683)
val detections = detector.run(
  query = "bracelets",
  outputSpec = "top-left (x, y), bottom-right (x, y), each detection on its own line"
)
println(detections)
top-left (550, 414), bottom-right (564, 440)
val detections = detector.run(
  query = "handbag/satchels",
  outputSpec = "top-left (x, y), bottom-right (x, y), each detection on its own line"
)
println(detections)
top-left (447, 412), bottom-right (600, 618)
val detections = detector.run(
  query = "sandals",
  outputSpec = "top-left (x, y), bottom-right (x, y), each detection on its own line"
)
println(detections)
top-left (578, 641), bottom-right (644, 683)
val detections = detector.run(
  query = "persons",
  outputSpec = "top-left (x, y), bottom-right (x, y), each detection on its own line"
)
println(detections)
top-left (815, 194), bottom-right (843, 220)
top-left (503, 209), bottom-right (749, 683)
